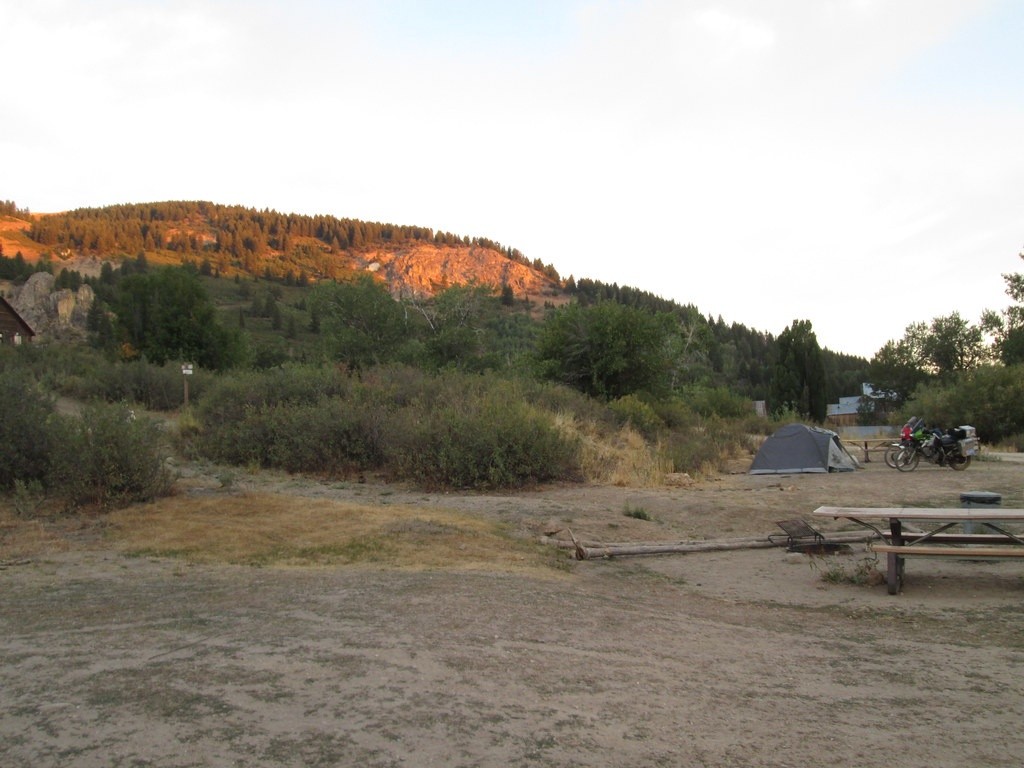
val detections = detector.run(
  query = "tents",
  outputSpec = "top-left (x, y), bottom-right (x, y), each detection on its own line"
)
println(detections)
top-left (749, 424), bottom-right (857, 474)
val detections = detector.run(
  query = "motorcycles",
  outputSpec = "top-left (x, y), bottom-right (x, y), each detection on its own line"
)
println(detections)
top-left (884, 416), bottom-right (981, 472)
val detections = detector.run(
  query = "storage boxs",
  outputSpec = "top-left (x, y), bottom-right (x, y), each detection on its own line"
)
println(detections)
top-left (956, 425), bottom-right (980, 457)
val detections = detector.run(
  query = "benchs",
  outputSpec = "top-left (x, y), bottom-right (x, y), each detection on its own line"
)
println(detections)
top-left (881, 532), bottom-right (1024, 572)
top-left (863, 447), bottom-right (900, 451)
top-left (870, 544), bottom-right (1024, 595)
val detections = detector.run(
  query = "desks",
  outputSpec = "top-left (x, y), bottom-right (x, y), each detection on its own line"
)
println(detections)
top-left (841, 438), bottom-right (903, 463)
top-left (813, 506), bottom-right (1024, 580)
